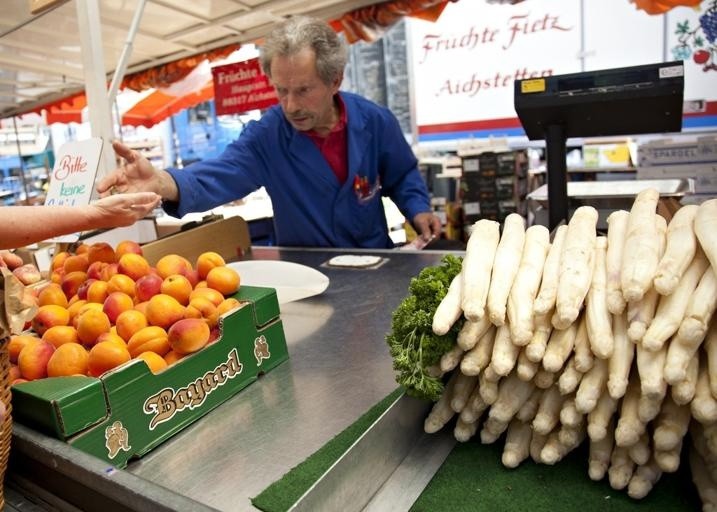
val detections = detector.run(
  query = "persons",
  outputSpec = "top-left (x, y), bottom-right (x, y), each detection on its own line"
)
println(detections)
top-left (0, 192), bottom-right (164, 250)
top-left (96, 16), bottom-right (441, 250)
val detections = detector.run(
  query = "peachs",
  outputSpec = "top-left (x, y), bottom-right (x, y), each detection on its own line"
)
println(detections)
top-left (0, 241), bottom-right (241, 390)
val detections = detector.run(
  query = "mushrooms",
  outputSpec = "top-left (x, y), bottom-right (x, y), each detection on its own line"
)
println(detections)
top-left (423, 189), bottom-right (717, 498)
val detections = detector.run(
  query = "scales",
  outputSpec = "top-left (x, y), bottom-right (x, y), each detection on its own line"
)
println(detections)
top-left (514, 61), bottom-right (695, 236)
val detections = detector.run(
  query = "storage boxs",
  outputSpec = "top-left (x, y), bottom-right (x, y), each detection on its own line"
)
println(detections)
top-left (565, 133), bottom-right (717, 192)
top-left (7, 280), bottom-right (292, 472)
top-left (409, 130), bottom-right (546, 248)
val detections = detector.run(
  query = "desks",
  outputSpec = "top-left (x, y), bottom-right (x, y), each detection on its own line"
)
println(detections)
top-left (4, 234), bottom-right (466, 509)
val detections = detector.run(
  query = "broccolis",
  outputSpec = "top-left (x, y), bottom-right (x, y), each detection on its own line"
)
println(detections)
top-left (388, 252), bottom-right (464, 406)
